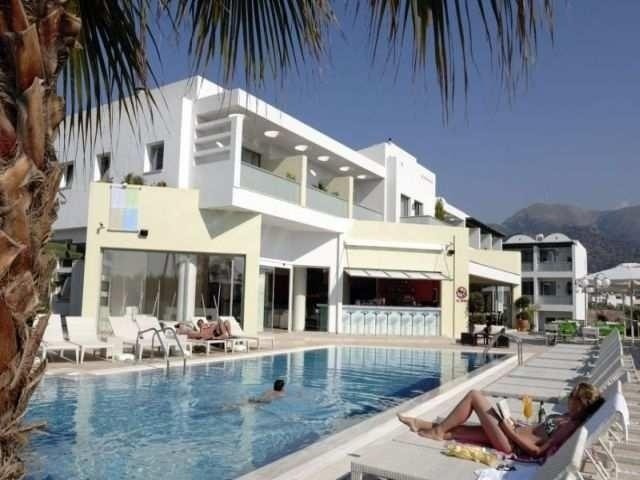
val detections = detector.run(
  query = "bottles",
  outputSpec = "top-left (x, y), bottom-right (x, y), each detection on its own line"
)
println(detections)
top-left (539, 400), bottom-right (545, 424)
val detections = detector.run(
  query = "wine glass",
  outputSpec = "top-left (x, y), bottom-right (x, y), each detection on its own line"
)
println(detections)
top-left (523, 397), bottom-right (532, 426)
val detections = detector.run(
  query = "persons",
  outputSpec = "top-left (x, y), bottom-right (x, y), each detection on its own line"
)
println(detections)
top-left (395, 380), bottom-right (600, 461)
top-left (197, 317), bottom-right (237, 341)
top-left (206, 314), bottom-right (217, 326)
top-left (174, 320), bottom-right (215, 340)
top-left (247, 378), bottom-right (288, 406)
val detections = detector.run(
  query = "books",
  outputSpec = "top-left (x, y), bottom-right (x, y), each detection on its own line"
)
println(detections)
top-left (495, 397), bottom-right (512, 422)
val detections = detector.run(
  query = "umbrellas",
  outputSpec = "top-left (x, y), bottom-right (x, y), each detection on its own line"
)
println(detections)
top-left (578, 279), bottom-right (640, 340)
top-left (574, 261), bottom-right (640, 346)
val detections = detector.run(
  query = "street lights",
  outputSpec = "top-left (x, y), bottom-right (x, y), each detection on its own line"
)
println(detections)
top-left (572, 273), bottom-right (612, 328)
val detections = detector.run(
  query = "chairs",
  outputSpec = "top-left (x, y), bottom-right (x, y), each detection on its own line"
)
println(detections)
top-left (30, 313), bottom-right (276, 369)
top-left (349, 320), bottom-right (629, 480)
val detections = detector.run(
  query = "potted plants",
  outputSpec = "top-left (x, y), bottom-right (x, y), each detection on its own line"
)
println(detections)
top-left (514, 294), bottom-right (531, 331)
